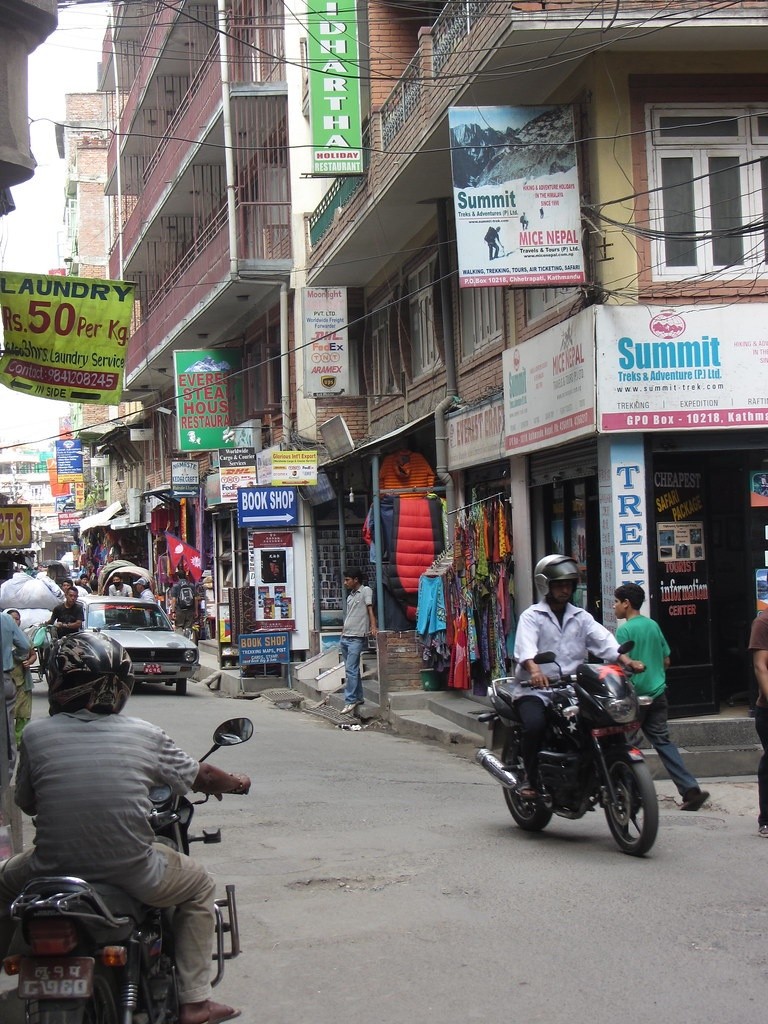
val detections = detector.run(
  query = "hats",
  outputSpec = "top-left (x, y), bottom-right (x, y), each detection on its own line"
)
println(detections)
top-left (133, 577), bottom-right (151, 586)
top-left (176, 569), bottom-right (186, 578)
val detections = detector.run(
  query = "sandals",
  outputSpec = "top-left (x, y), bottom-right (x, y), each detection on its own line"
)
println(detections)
top-left (758, 823), bottom-right (768, 838)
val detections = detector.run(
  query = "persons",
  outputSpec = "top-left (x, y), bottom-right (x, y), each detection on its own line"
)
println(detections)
top-left (514, 554), bottom-right (645, 800)
top-left (606, 584), bottom-right (709, 811)
top-left (340, 567), bottom-right (377, 715)
top-left (0, 632), bottom-right (250, 1024)
top-left (748, 609), bottom-right (768, 837)
top-left (0, 561), bottom-right (199, 786)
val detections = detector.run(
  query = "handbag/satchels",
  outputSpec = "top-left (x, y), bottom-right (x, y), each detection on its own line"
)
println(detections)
top-left (23, 666), bottom-right (35, 692)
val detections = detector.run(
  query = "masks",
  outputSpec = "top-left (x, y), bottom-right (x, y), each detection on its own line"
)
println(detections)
top-left (112, 580), bottom-right (121, 587)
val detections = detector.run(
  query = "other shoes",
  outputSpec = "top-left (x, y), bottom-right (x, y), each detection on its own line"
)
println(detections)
top-left (340, 697), bottom-right (365, 714)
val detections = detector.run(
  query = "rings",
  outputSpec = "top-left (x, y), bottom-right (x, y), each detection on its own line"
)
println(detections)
top-left (639, 664), bottom-right (643, 666)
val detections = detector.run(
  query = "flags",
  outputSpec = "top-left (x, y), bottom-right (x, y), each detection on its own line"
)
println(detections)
top-left (183, 543), bottom-right (204, 582)
top-left (165, 533), bottom-right (183, 583)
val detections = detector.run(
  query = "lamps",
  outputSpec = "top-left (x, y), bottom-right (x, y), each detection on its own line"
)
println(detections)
top-left (113, 420), bottom-right (132, 431)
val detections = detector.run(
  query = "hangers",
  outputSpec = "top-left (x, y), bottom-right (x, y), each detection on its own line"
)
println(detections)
top-left (421, 542), bottom-right (454, 576)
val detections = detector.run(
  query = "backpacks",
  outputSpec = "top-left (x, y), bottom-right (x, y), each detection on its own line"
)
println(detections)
top-left (175, 581), bottom-right (194, 608)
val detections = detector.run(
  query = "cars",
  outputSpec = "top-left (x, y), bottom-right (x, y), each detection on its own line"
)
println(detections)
top-left (77, 596), bottom-right (201, 695)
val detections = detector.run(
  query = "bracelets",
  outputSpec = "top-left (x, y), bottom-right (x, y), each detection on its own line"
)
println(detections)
top-left (229, 772), bottom-right (243, 794)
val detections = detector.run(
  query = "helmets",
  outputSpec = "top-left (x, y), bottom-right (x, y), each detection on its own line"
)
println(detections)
top-left (46, 630), bottom-right (134, 714)
top-left (533, 554), bottom-right (579, 597)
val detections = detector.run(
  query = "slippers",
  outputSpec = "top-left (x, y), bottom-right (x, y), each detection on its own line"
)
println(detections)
top-left (520, 781), bottom-right (544, 799)
top-left (679, 791), bottom-right (710, 813)
top-left (201, 1000), bottom-right (241, 1024)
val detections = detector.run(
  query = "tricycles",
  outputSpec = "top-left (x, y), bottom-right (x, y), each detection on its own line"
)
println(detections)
top-left (98, 560), bottom-right (156, 622)
top-left (30, 624), bottom-right (59, 686)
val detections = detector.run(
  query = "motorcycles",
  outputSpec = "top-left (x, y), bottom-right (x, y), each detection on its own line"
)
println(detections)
top-left (475, 640), bottom-right (660, 856)
top-left (2, 717), bottom-right (252, 1024)
